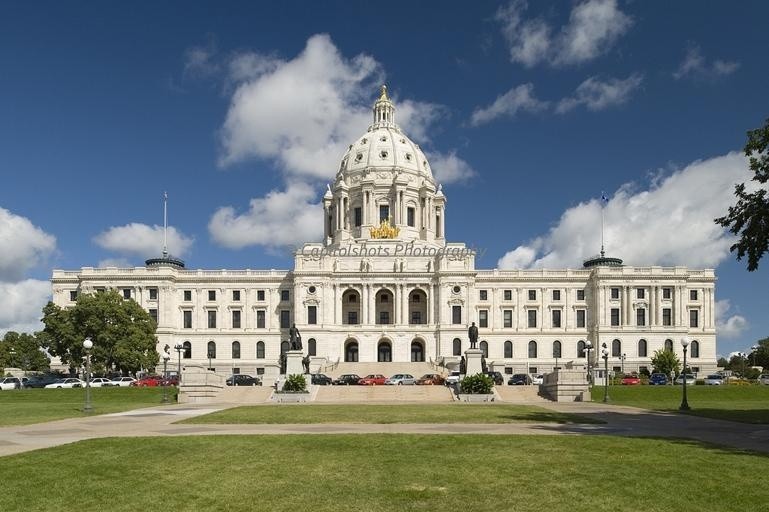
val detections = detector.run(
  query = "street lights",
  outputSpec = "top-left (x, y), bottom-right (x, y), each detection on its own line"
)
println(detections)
top-left (751, 345), bottom-right (759, 366)
top-left (618, 353), bottom-right (627, 373)
top-left (679, 336), bottom-right (691, 410)
top-left (582, 340), bottom-right (595, 382)
top-left (602, 348), bottom-right (610, 403)
top-left (82, 338), bottom-right (93, 414)
top-left (175, 344), bottom-right (188, 375)
top-left (162, 352), bottom-right (169, 404)
top-left (738, 351), bottom-right (747, 378)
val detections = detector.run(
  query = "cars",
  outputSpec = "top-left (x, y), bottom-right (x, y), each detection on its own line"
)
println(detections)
top-left (310, 372), bottom-right (461, 385)
top-left (0, 372), bottom-right (180, 391)
top-left (757, 374), bottom-right (769, 385)
top-left (483, 371), bottom-right (544, 385)
top-left (226, 374), bottom-right (260, 386)
top-left (621, 373), bottom-right (723, 386)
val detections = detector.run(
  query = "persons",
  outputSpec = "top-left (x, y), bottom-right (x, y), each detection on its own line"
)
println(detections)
top-left (288, 323), bottom-right (301, 350)
top-left (280, 354), bottom-right (285, 374)
top-left (482, 354), bottom-right (486, 370)
top-left (460, 356), bottom-right (466, 373)
top-left (468, 322), bottom-right (479, 348)
top-left (304, 354), bottom-right (310, 374)
